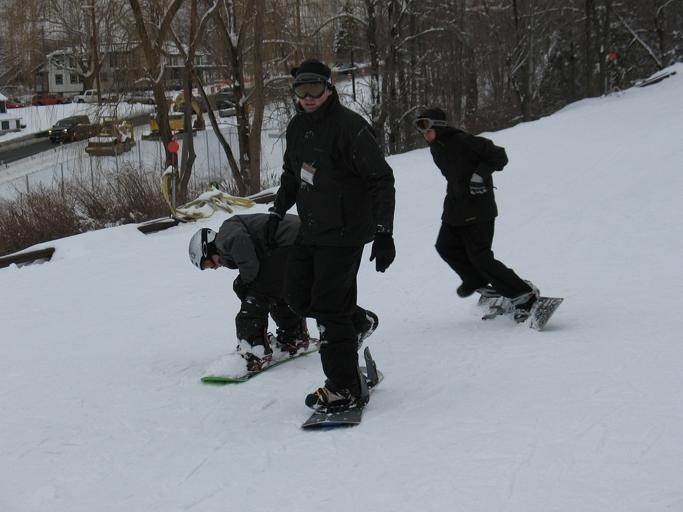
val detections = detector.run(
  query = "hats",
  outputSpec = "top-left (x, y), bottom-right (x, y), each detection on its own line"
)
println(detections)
top-left (296, 58), bottom-right (330, 83)
top-left (418, 107), bottom-right (445, 129)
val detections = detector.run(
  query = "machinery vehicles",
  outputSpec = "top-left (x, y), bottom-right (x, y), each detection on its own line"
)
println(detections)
top-left (84, 115), bottom-right (136, 156)
top-left (214, 86), bottom-right (254, 117)
top-left (141, 88), bottom-right (205, 142)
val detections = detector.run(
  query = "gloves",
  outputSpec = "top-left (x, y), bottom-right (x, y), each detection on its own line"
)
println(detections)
top-left (469, 170), bottom-right (488, 195)
top-left (263, 210), bottom-right (281, 249)
top-left (370, 235), bottom-right (395, 272)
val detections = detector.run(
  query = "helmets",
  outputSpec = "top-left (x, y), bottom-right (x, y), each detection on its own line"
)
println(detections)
top-left (189, 228), bottom-right (217, 270)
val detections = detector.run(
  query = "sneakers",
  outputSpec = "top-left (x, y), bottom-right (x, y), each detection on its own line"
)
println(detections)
top-left (237, 332), bottom-right (309, 370)
top-left (482, 280), bottom-right (540, 323)
top-left (356, 311), bottom-right (378, 349)
top-left (305, 385), bottom-right (368, 412)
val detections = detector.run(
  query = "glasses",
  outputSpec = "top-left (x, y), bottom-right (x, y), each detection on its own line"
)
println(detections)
top-left (413, 118), bottom-right (444, 131)
top-left (293, 80), bottom-right (326, 99)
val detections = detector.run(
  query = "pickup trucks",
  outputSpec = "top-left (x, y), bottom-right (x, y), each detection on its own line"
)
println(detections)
top-left (73, 89), bottom-right (119, 104)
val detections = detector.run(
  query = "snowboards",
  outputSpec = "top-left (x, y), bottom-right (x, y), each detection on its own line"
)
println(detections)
top-left (302, 366), bottom-right (383, 426)
top-left (201, 338), bottom-right (320, 383)
top-left (477, 295), bottom-right (563, 331)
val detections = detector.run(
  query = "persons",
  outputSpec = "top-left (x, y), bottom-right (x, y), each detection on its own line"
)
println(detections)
top-left (188, 212), bottom-right (379, 374)
top-left (413, 107), bottom-right (541, 324)
top-left (263, 58), bottom-right (396, 414)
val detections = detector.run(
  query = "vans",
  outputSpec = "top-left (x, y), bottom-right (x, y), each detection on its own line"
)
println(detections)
top-left (48, 114), bottom-right (90, 144)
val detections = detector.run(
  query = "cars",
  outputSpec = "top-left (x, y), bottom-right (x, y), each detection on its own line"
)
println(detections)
top-left (6, 99), bottom-right (25, 108)
top-left (123, 91), bottom-right (157, 105)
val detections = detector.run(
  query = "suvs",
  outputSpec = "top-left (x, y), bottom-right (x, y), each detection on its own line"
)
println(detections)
top-left (32, 93), bottom-right (65, 106)
